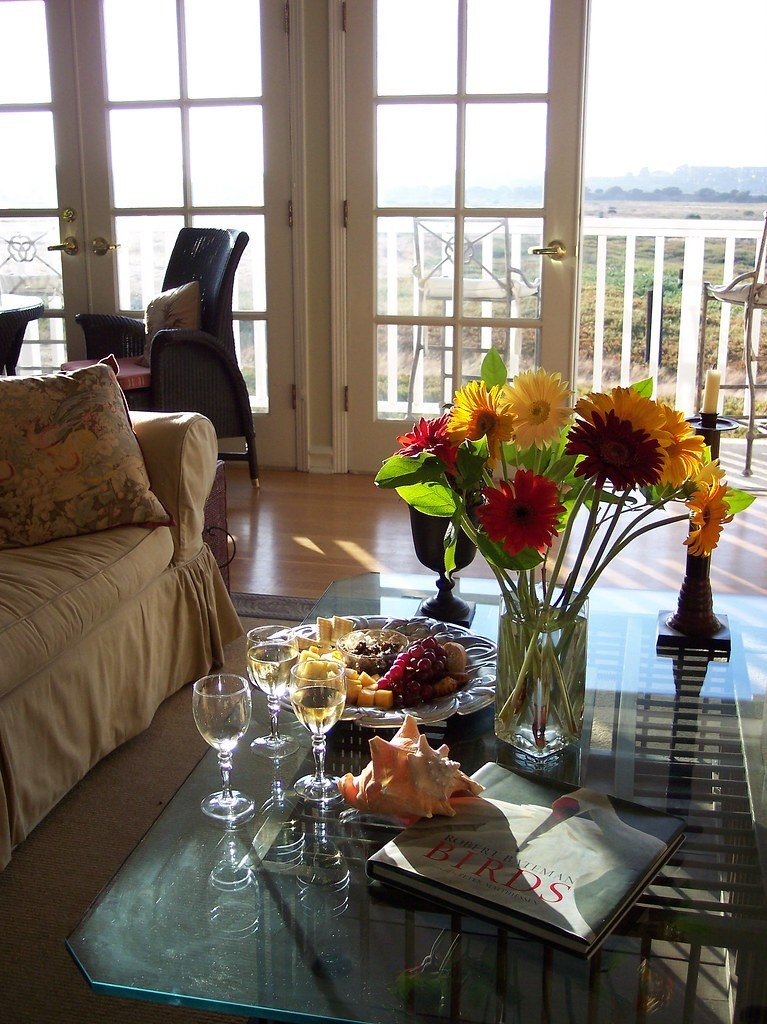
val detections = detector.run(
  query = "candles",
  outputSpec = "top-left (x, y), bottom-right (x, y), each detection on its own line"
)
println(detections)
top-left (699, 364), bottom-right (723, 415)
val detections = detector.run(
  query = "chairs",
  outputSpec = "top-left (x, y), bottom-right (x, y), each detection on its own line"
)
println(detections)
top-left (61, 227), bottom-right (261, 488)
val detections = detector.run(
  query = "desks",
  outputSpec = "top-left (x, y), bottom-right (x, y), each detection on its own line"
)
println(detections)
top-left (0, 293), bottom-right (45, 376)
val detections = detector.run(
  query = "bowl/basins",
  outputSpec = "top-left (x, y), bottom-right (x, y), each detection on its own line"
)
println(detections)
top-left (335, 629), bottom-right (410, 676)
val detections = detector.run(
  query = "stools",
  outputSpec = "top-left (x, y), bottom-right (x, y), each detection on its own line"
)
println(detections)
top-left (692, 210), bottom-right (767, 478)
top-left (403, 215), bottom-right (540, 422)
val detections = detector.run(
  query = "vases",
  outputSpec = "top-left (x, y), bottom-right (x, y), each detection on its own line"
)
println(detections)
top-left (493, 582), bottom-right (590, 761)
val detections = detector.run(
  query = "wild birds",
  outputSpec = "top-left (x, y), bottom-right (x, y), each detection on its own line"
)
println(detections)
top-left (384, 787), bottom-right (668, 944)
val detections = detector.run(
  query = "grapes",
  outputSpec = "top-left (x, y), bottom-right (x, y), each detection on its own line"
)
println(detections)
top-left (378, 636), bottom-right (447, 707)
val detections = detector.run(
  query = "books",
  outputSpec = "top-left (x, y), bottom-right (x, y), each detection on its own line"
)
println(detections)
top-left (364, 777), bottom-right (688, 961)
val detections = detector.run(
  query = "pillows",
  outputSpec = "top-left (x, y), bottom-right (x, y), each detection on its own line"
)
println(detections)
top-left (135, 280), bottom-right (200, 369)
top-left (0, 352), bottom-right (179, 549)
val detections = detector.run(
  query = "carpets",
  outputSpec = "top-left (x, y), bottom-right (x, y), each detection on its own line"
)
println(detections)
top-left (0, 594), bottom-right (767, 1024)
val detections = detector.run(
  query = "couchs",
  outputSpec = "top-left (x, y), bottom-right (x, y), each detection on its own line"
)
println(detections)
top-left (0, 410), bottom-right (246, 868)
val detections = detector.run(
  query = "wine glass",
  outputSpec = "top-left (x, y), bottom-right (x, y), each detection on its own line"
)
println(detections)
top-left (286, 659), bottom-right (348, 802)
top-left (190, 672), bottom-right (255, 821)
top-left (244, 624), bottom-right (299, 759)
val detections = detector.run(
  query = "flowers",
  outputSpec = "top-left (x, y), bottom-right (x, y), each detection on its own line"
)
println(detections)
top-left (373, 344), bottom-right (757, 748)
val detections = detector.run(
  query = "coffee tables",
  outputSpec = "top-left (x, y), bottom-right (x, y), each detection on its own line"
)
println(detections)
top-left (65, 571), bottom-right (766, 1023)
